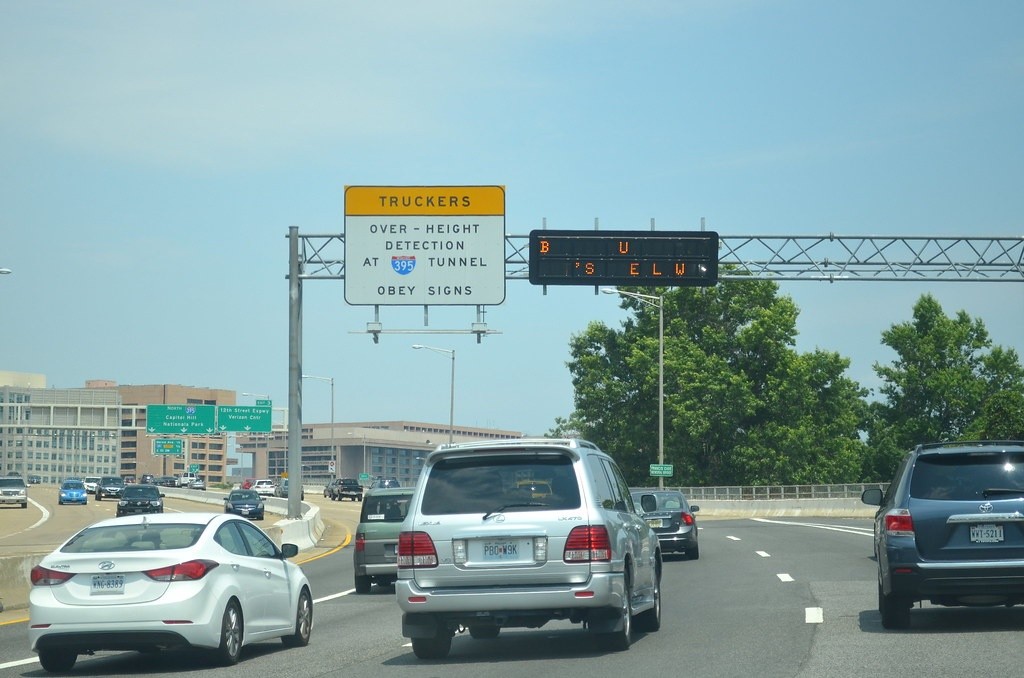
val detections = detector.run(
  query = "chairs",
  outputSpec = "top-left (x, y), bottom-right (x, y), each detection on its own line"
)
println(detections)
top-left (381, 509), bottom-right (400, 518)
top-left (665, 501), bottom-right (680, 508)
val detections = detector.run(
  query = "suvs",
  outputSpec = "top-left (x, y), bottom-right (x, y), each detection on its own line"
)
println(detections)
top-left (0, 477), bottom-right (31, 507)
top-left (95, 477), bottom-right (125, 500)
top-left (860, 441), bottom-right (1024, 630)
top-left (116, 483), bottom-right (165, 516)
top-left (394, 439), bottom-right (664, 659)
top-left (353, 487), bottom-right (416, 593)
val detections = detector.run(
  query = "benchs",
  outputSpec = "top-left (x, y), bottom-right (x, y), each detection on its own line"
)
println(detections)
top-left (82, 534), bottom-right (192, 548)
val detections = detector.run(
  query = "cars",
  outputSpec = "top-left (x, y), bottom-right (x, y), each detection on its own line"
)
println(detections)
top-left (323, 476), bottom-right (401, 502)
top-left (30, 511), bottom-right (315, 673)
top-left (223, 490), bottom-right (266, 520)
top-left (632, 490), bottom-right (700, 561)
top-left (58, 480), bottom-right (88, 505)
top-left (82, 472), bottom-right (206, 493)
top-left (28, 475), bottom-right (43, 484)
top-left (239, 477), bottom-right (305, 501)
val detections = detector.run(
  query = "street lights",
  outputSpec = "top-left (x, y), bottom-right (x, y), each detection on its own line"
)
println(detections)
top-left (302, 374), bottom-right (335, 481)
top-left (601, 287), bottom-right (664, 489)
top-left (241, 392), bottom-right (270, 401)
top-left (411, 344), bottom-right (455, 444)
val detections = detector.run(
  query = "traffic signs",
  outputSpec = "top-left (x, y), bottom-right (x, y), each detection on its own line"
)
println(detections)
top-left (217, 405), bottom-right (271, 432)
top-left (145, 404), bottom-right (216, 435)
